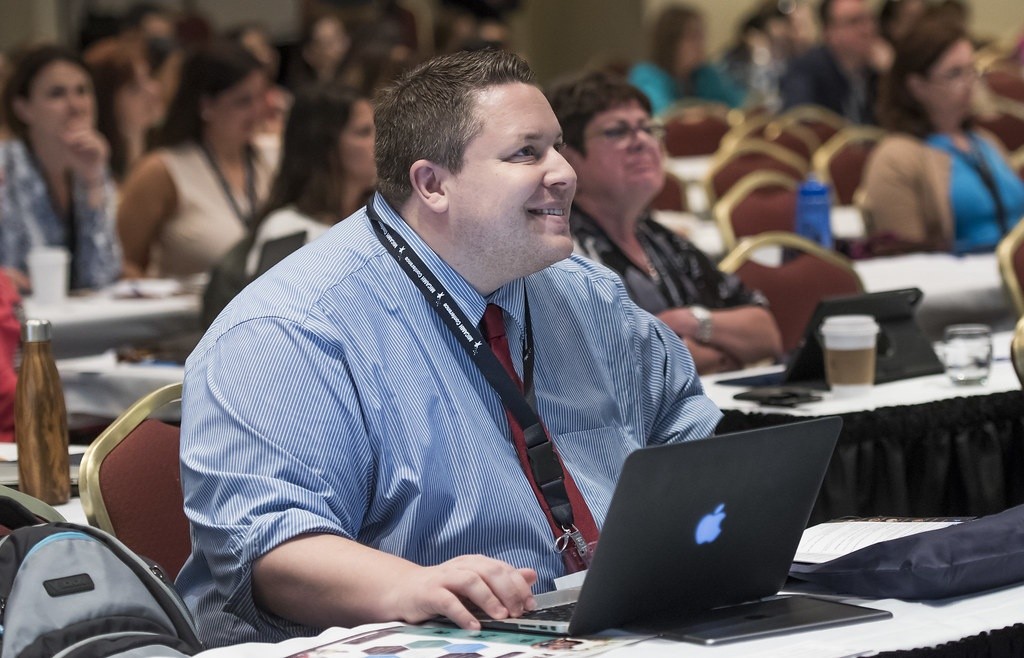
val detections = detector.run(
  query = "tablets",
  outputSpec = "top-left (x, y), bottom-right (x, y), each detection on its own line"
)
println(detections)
top-left (781, 288), bottom-right (924, 383)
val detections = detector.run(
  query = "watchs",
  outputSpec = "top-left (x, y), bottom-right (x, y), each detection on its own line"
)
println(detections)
top-left (692, 306), bottom-right (714, 343)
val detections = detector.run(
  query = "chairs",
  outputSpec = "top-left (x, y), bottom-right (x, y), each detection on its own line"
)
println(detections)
top-left (994, 217), bottom-right (1024, 318)
top-left (763, 106), bottom-right (855, 168)
top-left (713, 169), bottom-right (801, 256)
top-left (720, 113), bottom-right (776, 150)
top-left (78, 382), bottom-right (193, 586)
top-left (969, 41), bottom-right (1024, 182)
top-left (658, 100), bottom-right (733, 159)
top-left (811, 125), bottom-right (888, 205)
top-left (716, 230), bottom-right (866, 356)
top-left (651, 171), bottom-right (689, 212)
top-left (701, 138), bottom-right (807, 209)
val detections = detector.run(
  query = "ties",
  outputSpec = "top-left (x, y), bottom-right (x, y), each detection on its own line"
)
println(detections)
top-left (474, 304), bottom-right (600, 575)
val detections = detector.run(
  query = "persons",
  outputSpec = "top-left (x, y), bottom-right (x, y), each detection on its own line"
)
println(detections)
top-left (170, 47), bottom-right (725, 645)
top-left (552, 71), bottom-right (785, 373)
top-left (1, 0), bottom-right (1024, 334)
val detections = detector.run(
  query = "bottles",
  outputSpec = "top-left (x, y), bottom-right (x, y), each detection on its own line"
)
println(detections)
top-left (14, 317), bottom-right (72, 507)
top-left (793, 179), bottom-right (834, 250)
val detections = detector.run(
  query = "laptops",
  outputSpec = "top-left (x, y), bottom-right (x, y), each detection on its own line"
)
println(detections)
top-left (431, 416), bottom-right (845, 637)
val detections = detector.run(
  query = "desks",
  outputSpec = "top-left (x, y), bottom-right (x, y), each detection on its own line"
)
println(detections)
top-left (851, 251), bottom-right (1018, 343)
top-left (187, 520), bottom-right (1024, 658)
top-left (651, 205), bottom-right (866, 266)
top-left (698, 328), bottom-right (1023, 517)
top-left (21, 269), bottom-right (212, 358)
top-left (52, 332), bottom-right (204, 420)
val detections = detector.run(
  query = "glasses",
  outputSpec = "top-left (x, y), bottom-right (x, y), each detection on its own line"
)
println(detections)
top-left (581, 117), bottom-right (668, 147)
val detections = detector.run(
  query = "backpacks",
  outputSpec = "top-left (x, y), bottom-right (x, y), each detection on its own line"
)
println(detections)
top-left (0, 486), bottom-right (208, 658)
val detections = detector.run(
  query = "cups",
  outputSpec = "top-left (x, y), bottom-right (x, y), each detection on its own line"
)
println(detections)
top-left (25, 245), bottom-right (69, 319)
top-left (819, 314), bottom-right (880, 394)
top-left (943, 323), bottom-right (993, 382)
